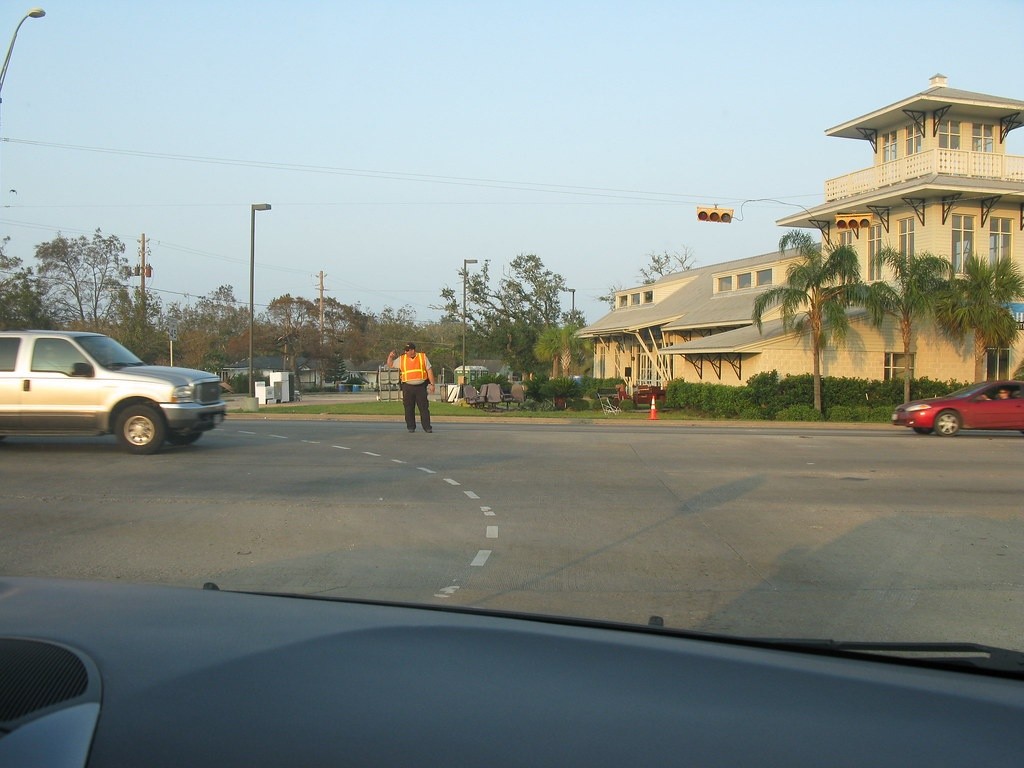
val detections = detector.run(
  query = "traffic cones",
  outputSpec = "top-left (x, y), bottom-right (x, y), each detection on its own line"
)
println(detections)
top-left (645, 396), bottom-right (661, 420)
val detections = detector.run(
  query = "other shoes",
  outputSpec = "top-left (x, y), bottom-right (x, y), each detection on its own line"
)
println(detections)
top-left (424, 426), bottom-right (433, 433)
top-left (407, 428), bottom-right (416, 433)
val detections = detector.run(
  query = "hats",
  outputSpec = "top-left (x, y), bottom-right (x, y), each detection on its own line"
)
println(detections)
top-left (403, 342), bottom-right (416, 350)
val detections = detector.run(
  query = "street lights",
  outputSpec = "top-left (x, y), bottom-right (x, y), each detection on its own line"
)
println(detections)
top-left (567, 288), bottom-right (575, 313)
top-left (243, 203), bottom-right (271, 413)
top-left (461, 258), bottom-right (477, 377)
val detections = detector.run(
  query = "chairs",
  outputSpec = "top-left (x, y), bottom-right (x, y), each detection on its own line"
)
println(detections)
top-left (464, 384), bottom-right (524, 413)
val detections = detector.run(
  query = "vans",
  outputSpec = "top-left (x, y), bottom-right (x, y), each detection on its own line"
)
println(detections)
top-left (454, 366), bottom-right (490, 386)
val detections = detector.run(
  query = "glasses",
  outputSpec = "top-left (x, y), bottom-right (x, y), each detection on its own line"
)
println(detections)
top-left (406, 350), bottom-right (409, 351)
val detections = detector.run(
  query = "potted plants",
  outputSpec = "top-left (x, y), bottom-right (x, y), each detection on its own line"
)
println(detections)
top-left (539, 375), bottom-right (584, 410)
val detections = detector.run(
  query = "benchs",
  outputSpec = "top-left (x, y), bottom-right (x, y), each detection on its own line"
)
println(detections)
top-left (596, 387), bottom-right (618, 399)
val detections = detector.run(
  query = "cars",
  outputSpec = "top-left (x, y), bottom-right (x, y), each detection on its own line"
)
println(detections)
top-left (892, 379), bottom-right (1024, 437)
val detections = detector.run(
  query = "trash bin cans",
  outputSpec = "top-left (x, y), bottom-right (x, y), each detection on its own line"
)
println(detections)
top-left (352, 385), bottom-right (362, 392)
top-left (339, 384), bottom-right (346, 392)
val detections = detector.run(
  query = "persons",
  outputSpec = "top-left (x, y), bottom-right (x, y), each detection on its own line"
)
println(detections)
top-left (40, 344), bottom-right (65, 371)
top-left (388, 343), bottom-right (436, 433)
top-left (616, 382), bottom-right (627, 400)
top-left (980, 386), bottom-right (1011, 401)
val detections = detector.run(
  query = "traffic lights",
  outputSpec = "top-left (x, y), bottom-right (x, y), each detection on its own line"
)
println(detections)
top-left (697, 207), bottom-right (734, 224)
top-left (834, 213), bottom-right (874, 229)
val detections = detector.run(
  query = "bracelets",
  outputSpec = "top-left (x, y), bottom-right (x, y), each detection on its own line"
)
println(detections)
top-left (431, 383), bottom-right (435, 386)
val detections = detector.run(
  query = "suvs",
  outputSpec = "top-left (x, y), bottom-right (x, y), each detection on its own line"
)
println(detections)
top-left (0, 324), bottom-right (226, 456)
